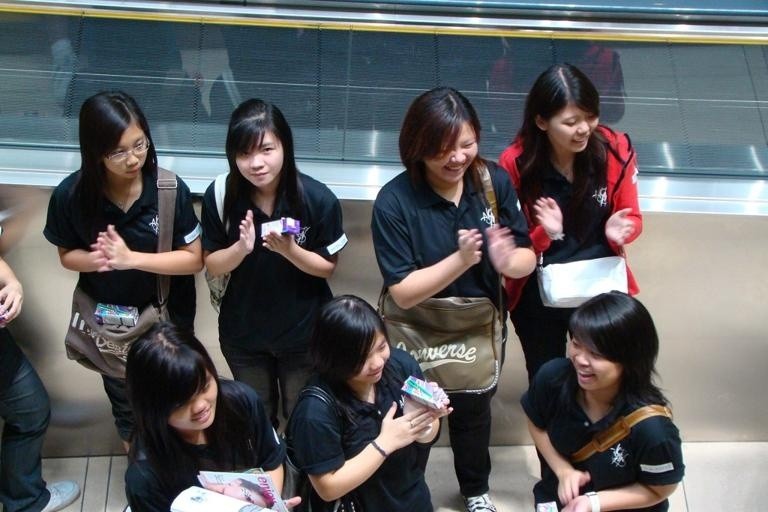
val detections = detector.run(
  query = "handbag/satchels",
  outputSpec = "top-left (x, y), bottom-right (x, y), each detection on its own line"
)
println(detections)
top-left (64, 286), bottom-right (170, 380)
top-left (376, 292), bottom-right (503, 395)
top-left (280, 384), bottom-right (364, 512)
top-left (205, 170), bottom-right (230, 314)
top-left (537, 255), bottom-right (628, 309)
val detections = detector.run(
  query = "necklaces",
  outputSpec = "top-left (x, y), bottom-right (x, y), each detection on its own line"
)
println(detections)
top-left (560, 163), bottom-right (574, 178)
top-left (113, 199), bottom-right (132, 211)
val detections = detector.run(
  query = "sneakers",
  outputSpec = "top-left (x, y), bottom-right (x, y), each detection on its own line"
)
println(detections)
top-left (463, 489), bottom-right (496, 512)
top-left (42, 481), bottom-right (80, 510)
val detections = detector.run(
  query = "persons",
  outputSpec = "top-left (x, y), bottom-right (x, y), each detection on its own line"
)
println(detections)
top-left (499, 64), bottom-right (642, 386)
top-left (200, 99), bottom-right (348, 429)
top-left (284, 294), bottom-right (453, 512)
top-left (519, 291), bottom-right (685, 511)
top-left (0, 227), bottom-right (81, 512)
top-left (371, 88), bottom-right (538, 512)
top-left (124, 327), bottom-right (301, 512)
top-left (29, 16), bottom-right (629, 163)
top-left (44, 90), bottom-right (204, 452)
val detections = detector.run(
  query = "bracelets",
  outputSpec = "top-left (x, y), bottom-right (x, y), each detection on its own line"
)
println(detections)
top-left (584, 490), bottom-right (600, 511)
top-left (545, 233), bottom-right (565, 242)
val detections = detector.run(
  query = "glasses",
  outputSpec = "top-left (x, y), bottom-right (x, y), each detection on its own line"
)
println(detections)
top-left (105, 135), bottom-right (151, 163)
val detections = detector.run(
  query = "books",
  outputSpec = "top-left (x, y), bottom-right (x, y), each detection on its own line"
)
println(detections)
top-left (170, 465), bottom-right (290, 512)
top-left (372, 441), bottom-right (388, 459)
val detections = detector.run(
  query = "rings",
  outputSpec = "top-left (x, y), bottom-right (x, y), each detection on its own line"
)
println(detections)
top-left (409, 420), bottom-right (416, 430)
